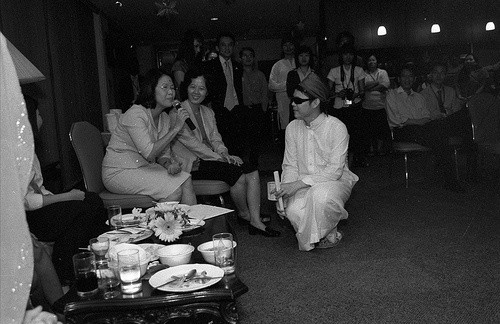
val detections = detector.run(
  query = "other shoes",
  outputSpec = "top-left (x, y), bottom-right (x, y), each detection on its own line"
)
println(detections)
top-left (237, 213), bottom-right (271, 225)
top-left (248, 222), bottom-right (281, 237)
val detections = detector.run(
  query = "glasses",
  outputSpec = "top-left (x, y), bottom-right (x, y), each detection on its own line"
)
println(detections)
top-left (290, 96), bottom-right (311, 105)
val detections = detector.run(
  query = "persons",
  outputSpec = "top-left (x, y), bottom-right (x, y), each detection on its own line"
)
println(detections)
top-left (239, 47), bottom-right (270, 150)
top-left (319, 45), bottom-right (369, 168)
top-left (20, 96), bottom-right (109, 324)
top-left (203, 33), bottom-right (247, 150)
top-left (169, 68), bottom-right (281, 237)
top-left (101, 69), bottom-right (200, 204)
top-left (359, 54), bottom-right (391, 155)
top-left (269, 36), bottom-right (303, 159)
top-left (385, 53), bottom-right (500, 187)
top-left (286, 45), bottom-right (319, 121)
top-left (276, 72), bottom-right (359, 252)
top-left (164, 30), bottom-right (204, 99)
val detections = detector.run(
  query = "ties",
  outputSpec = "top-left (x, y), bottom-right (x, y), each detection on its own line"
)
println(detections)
top-left (437, 90), bottom-right (446, 113)
top-left (224, 61), bottom-right (234, 101)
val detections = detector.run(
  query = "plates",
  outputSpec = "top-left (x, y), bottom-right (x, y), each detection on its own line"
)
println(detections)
top-left (148, 263), bottom-right (225, 292)
top-left (97, 227), bottom-right (153, 244)
top-left (145, 204), bottom-right (190, 218)
top-left (148, 219), bottom-right (205, 232)
top-left (105, 243), bottom-right (165, 265)
top-left (105, 213), bottom-right (158, 227)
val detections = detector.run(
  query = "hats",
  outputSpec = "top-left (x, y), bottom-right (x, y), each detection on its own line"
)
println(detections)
top-left (298, 72), bottom-right (331, 117)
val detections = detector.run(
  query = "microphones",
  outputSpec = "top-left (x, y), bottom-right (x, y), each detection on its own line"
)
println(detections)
top-left (172, 100), bottom-right (196, 131)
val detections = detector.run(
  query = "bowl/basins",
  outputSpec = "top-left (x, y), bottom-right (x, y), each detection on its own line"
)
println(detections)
top-left (157, 244), bottom-right (195, 267)
top-left (197, 239), bottom-right (237, 264)
top-left (156, 201), bottom-right (179, 211)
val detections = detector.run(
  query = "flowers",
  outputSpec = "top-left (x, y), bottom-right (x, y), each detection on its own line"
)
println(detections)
top-left (132, 200), bottom-right (206, 245)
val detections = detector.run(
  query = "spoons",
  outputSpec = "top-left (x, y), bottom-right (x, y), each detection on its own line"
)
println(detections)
top-left (152, 269), bottom-right (196, 291)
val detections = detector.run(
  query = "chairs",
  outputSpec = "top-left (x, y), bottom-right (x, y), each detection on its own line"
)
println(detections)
top-left (191, 179), bottom-right (230, 206)
top-left (466, 92), bottom-right (500, 182)
top-left (33, 241), bottom-right (64, 304)
top-left (70, 120), bottom-right (156, 214)
top-left (388, 127), bottom-right (436, 189)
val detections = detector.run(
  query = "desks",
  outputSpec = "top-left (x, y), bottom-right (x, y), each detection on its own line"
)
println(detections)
top-left (53, 214), bottom-right (249, 324)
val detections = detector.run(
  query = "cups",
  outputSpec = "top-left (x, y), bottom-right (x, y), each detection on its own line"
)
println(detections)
top-left (170, 151), bottom-right (184, 167)
top-left (72, 252), bottom-right (99, 297)
top-left (117, 249), bottom-right (143, 294)
top-left (212, 232), bottom-right (233, 275)
top-left (108, 204), bottom-right (122, 230)
top-left (90, 237), bottom-right (110, 269)
top-left (98, 262), bottom-right (121, 300)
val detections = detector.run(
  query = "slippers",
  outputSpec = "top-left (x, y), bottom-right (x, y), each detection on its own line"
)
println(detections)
top-left (316, 229), bottom-right (344, 249)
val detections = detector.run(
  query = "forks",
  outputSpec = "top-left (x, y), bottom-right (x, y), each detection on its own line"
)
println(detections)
top-left (171, 276), bottom-right (223, 281)
